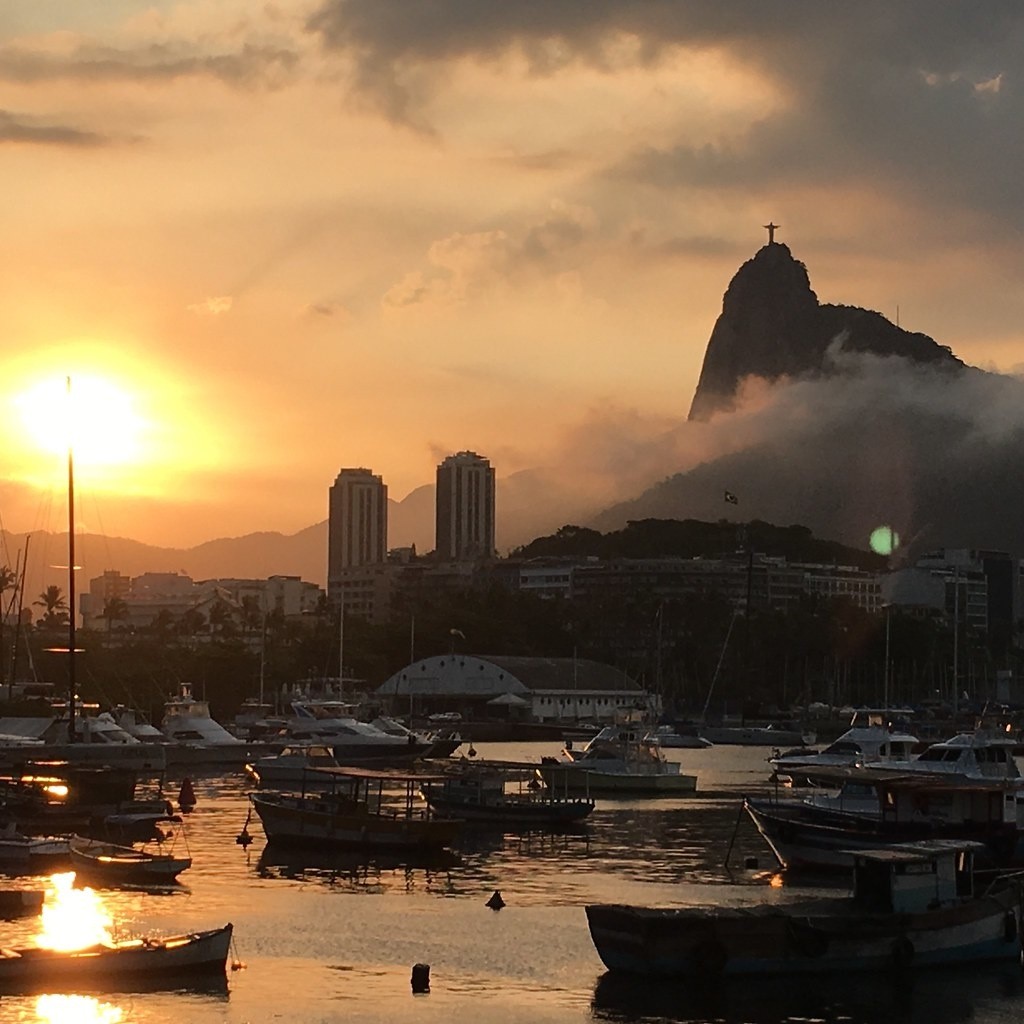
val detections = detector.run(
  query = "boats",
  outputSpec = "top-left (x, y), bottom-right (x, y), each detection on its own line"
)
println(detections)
top-left (534, 704), bottom-right (700, 799)
top-left (2, 922), bottom-right (245, 982)
top-left (639, 729), bottom-right (714, 751)
top-left (586, 839), bottom-right (1024, 1023)
top-left (0, 888), bottom-right (47, 909)
top-left (242, 763), bottom-right (472, 849)
top-left (416, 755), bottom-right (598, 826)
top-left (69, 833), bottom-right (194, 884)
top-left (1, 531), bottom-right (468, 863)
top-left (733, 540), bottom-right (1024, 882)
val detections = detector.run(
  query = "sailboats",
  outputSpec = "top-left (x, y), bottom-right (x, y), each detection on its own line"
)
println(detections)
top-left (557, 641), bottom-right (608, 741)
